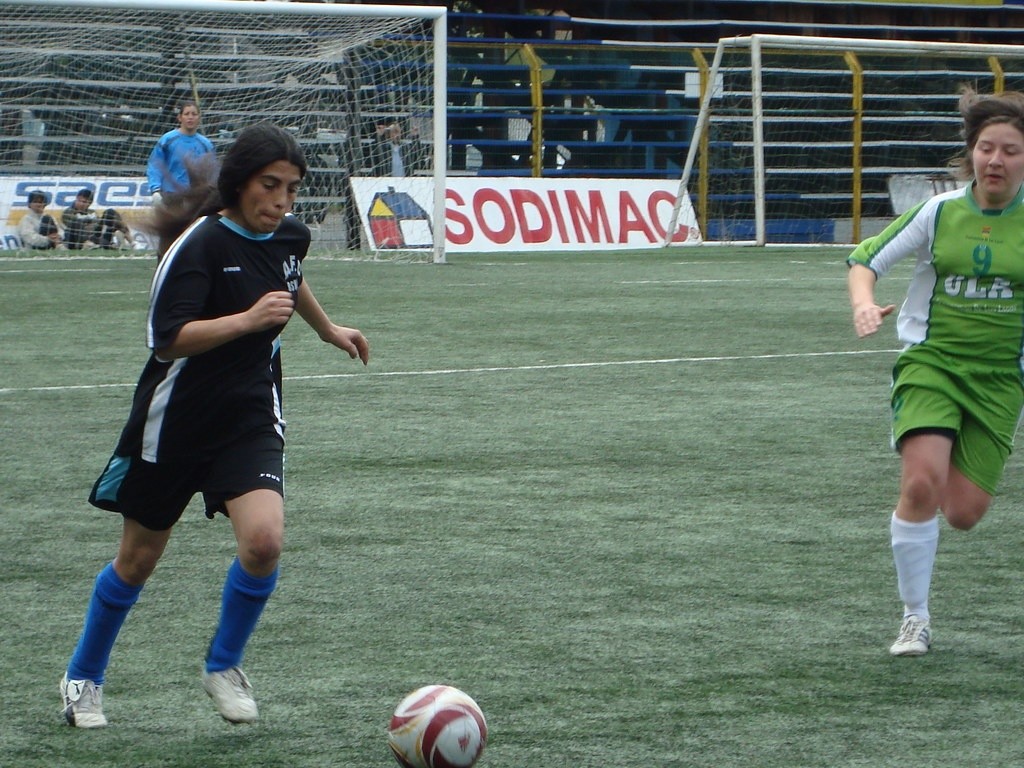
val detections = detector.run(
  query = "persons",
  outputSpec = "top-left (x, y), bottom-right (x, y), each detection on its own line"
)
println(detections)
top-left (146, 102), bottom-right (217, 262)
top-left (373, 124), bottom-right (420, 176)
top-left (61, 189), bottom-right (149, 251)
top-left (19, 189), bottom-right (69, 251)
top-left (843, 85), bottom-right (1023, 657)
top-left (61, 123), bottom-right (369, 728)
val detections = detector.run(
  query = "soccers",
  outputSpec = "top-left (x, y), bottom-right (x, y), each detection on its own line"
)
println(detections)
top-left (388, 685), bottom-right (488, 768)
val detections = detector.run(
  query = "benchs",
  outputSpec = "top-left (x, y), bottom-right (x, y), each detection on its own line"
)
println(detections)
top-left (5, 2), bottom-right (1023, 246)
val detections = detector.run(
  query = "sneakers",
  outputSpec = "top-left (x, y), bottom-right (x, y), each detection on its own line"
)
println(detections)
top-left (202, 667), bottom-right (258, 722)
top-left (890, 613), bottom-right (932, 656)
top-left (59, 671), bottom-right (107, 728)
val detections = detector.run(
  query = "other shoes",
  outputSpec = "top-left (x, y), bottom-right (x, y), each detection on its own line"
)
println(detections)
top-left (54, 241), bottom-right (149, 251)
